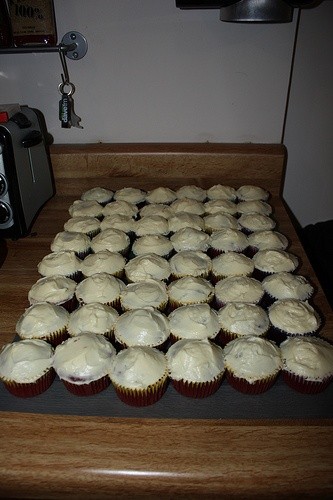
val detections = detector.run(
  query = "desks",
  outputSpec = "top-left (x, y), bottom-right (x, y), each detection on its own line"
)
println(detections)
top-left (0, 143), bottom-right (333, 500)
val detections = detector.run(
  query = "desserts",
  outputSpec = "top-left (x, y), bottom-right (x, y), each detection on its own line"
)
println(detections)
top-left (0, 184), bottom-right (333, 407)
